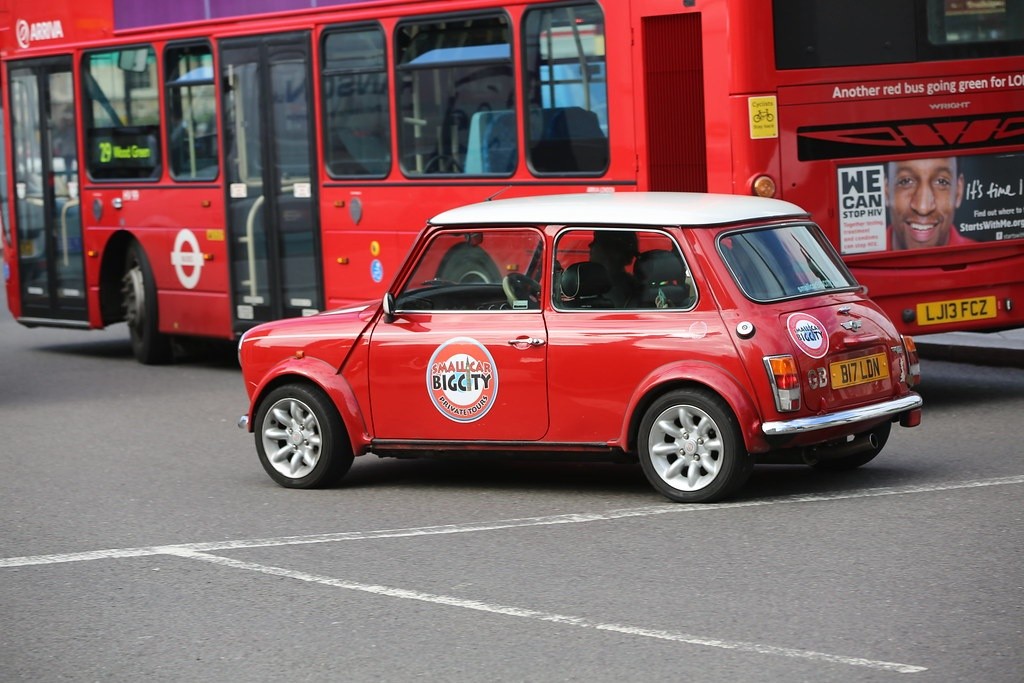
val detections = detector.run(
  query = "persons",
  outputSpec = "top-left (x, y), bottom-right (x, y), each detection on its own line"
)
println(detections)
top-left (884, 156), bottom-right (977, 251)
top-left (588, 230), bottom-right (638, 278)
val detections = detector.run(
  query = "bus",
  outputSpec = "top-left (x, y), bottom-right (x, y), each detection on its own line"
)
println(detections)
top-left (1, 0), bottom-right (1024, 364)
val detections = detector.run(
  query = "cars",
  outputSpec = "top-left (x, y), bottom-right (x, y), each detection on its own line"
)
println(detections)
top-left (237, 184), bottom-right (924, 504)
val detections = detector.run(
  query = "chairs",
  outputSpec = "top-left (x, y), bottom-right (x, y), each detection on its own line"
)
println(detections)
top-left (559, 262), bottom-right (615, 309)
top-left (466, 106), bottom-right (605, 174)
top-left (631, 248), bottom-right (691, 305)
